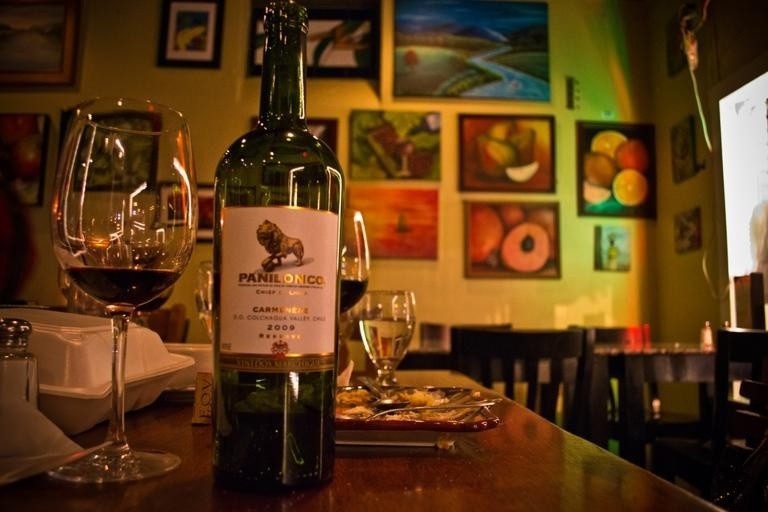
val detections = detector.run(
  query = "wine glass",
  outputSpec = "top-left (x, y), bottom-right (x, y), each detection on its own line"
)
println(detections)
top-left (194, 259), bottom-right (213, 345)
top-left (338, 207), bottom-right (371, 385)
top-left (41, 99), bottom-right (200, 485)
top-left (357, 289), bottom-right (416, 386)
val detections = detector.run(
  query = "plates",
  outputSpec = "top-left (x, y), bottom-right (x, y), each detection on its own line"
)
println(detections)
top-left (334, 384), bottom-right (501, 431)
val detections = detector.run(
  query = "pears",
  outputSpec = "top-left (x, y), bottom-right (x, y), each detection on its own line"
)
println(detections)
top-left (476, 121), bottom-right (539, 183)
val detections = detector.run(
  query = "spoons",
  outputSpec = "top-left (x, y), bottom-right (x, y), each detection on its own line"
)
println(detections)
top-left (354, 376), bottom-right (410, 411)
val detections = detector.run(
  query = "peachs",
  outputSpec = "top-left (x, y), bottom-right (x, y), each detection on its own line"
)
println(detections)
top-left (501, 223), bottom-right (548, 272)
top-left (615, 140), bottom-right (647, 174)
top-left (502, 206), bottom-right (525, 227)
top-left (529, 209), bottom-right (555, 239)
top-left (584, 151), bottom-right (616, 187)
top-left (471, 204), bottom-right (501, 264)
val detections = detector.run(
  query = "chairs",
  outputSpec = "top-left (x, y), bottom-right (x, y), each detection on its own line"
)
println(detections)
top-left (148, 304), bottom-right (190, 343)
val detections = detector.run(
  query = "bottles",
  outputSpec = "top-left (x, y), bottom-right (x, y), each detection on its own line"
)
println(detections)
top-left (209, 0), bottom-right (345, 497)
top-left (0, 317), bottom-right (38, 407)
top-left (700, 320), bottom-right (713, 348)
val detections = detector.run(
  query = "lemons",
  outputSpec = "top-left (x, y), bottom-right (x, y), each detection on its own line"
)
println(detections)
top-left (591, 131), bottom-right (626, 159)
top-left (613, 169), bottom-right (648, 206)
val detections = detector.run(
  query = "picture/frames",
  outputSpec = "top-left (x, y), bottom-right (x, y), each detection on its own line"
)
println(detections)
top-left (673, 206), bottom-right (700, 254)
top-left (670, 115), bottom-right (704, 183)
